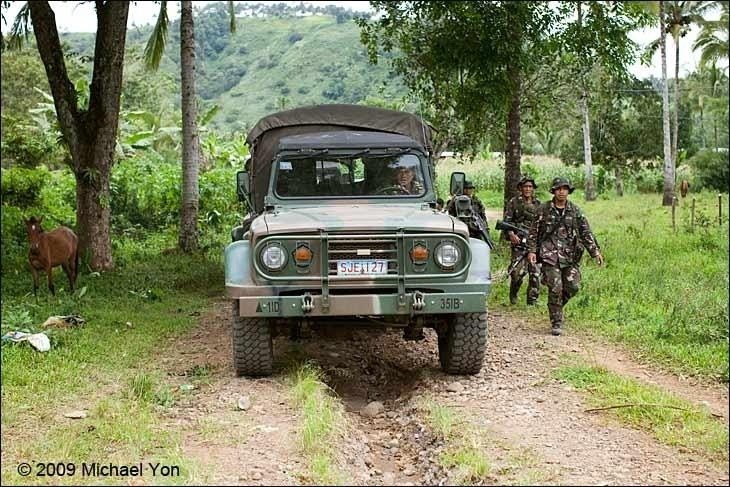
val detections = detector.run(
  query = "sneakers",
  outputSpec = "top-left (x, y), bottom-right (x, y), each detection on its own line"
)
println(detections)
top-left (510, 289), bottom-right (562, 336)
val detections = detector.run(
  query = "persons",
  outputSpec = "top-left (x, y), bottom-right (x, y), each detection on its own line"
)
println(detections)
top-left (448, 179), bottom-right (489, 242)
top-left (502, 176), bottom-right (543, 306)
top-left (526, 176), bottom-right (604, 337)
top-left (368, 157), bottom-right (426, 197)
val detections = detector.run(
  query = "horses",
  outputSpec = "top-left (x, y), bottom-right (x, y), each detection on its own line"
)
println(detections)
top-left (23, 215), bottom-right (78, 296)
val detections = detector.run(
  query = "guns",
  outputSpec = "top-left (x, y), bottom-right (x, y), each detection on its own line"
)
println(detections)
top-left (505, 239), bottom-right (540, 280)
top-left (494, 219), bottom-right (531, 246)
top-left (468, 206), bottom-right (496, 251)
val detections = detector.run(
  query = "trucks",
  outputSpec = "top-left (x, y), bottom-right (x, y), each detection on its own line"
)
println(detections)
top-left (224, 104), bottom-right (491, 375)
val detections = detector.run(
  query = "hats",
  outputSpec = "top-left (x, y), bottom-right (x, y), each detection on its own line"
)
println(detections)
top-left (464, 180), bottom-right (475, 189)
top-left (516, 176), bottom-right (538, 189)
top-left (548, 178), bottom-right (575, 193)
